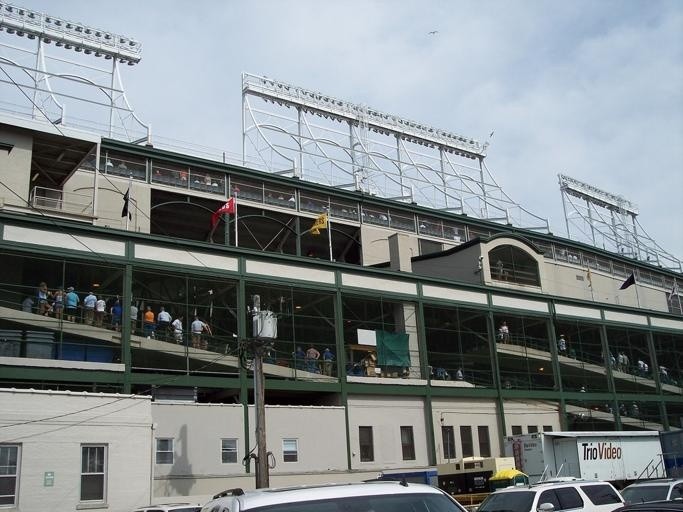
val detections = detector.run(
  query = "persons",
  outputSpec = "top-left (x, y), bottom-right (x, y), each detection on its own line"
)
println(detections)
top-left (104, 157), bottom-right (223, 190)
top-left (443, 367), bottom-right (464, 381)
top-left (22, 281), bottom-right (336, 382)
top-left (496, 320), bottom-right (679, 418)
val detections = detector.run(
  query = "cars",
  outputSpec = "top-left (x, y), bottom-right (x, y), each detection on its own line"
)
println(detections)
top-left (472, 473), bottom-right (682, 512)
top-left (132, 503), bottom-right (203, 512)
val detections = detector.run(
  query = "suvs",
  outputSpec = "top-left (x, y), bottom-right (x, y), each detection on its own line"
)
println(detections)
top-left (201, 479), bottom-right (469, 512)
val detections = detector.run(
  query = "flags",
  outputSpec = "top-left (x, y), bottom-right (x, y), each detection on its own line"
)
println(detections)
top-left (122, 188), bottom-right (132, 220)
top-left (668, 282), bottom-right (678, 299)
top-left (619, 274), bottom-right (635, 290)
top-left (310, 212), bottom-right (328, 234)
top-left (212, 197), bottom-right (235, 226)
